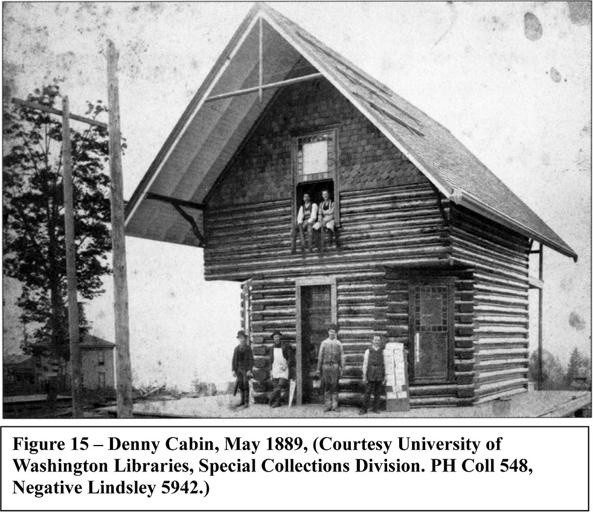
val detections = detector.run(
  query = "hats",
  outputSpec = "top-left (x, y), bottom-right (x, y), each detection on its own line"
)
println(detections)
top-left (270, 330), bottom-right (281, 338)
top-left (236, 330), bottom-right (247, 338)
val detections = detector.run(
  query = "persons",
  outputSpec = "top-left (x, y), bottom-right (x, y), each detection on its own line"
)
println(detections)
top-left (311, 188), bottom-right (334, 252)
top-left (314, 323), bottom-right (345, 410)
top-left (289, 192), bottom-right (317, 254)
top-left (230, 329), bottom-right (254, 408)
top-left (266, 329), bottom-right (292, 407)
top-left (356, 332), bottom-right (385, 415)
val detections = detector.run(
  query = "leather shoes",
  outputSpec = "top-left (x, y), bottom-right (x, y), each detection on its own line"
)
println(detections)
top-left (235, 398), bottom-right (381, 415)
top-left (289, 242), bottom-right (335, 253)
